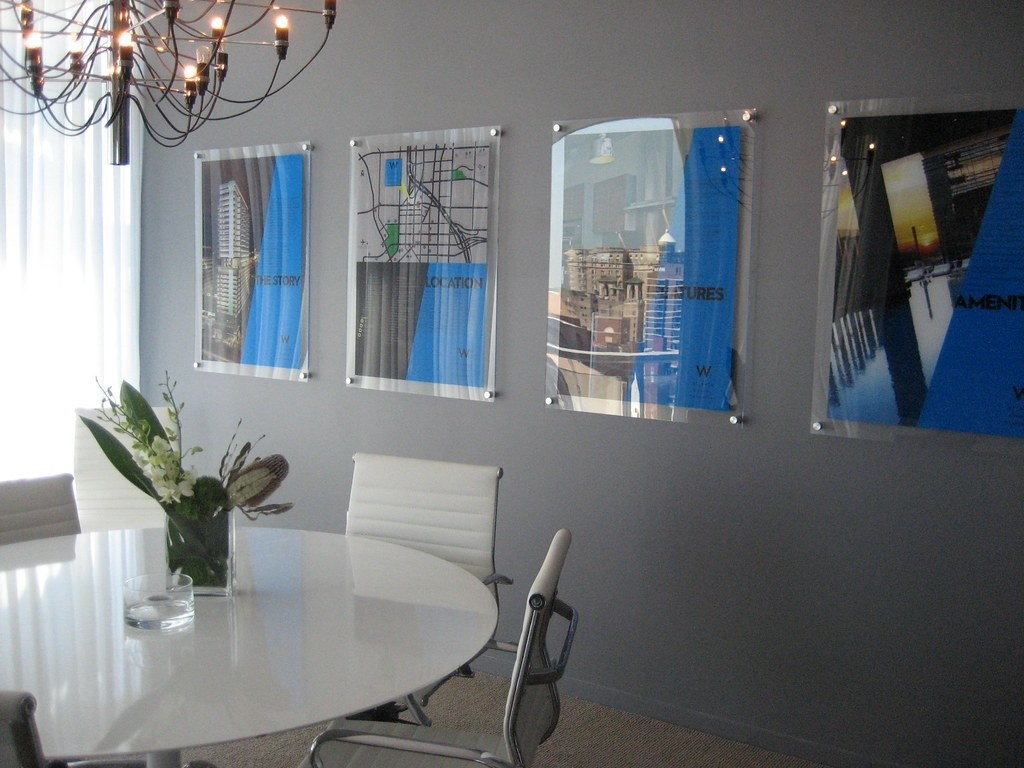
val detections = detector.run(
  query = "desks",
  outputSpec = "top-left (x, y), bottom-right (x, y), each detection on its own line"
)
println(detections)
top-left (0, 527), bottom-right (498, 768)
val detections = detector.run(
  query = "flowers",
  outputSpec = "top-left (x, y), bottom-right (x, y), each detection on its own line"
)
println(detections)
top-left (81, 369), bottom-right (295, 521)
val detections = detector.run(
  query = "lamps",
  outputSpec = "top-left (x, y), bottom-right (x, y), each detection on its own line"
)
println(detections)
top-left (0, 0), bottom-right (337, 166)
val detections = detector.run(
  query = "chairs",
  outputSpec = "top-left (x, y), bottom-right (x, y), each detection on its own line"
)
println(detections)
top-left (74, 406), bottom-right (180, 532)
top-left (345, 453), bottom-right (511, 712)
top-left (296, 526), bottom-right (578, 768)
top-left (0, 473), bottom-right (81, 545)
top-left (0, 689), bottom-right (216, 768)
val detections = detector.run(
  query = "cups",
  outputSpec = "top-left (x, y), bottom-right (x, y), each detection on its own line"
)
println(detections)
top-left (123, 570), bottom-right (196, 632)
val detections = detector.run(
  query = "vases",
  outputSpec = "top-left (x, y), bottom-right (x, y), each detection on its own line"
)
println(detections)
top-left (164, 507), bottom-right (239, 597)
